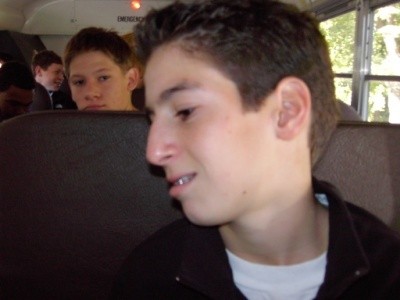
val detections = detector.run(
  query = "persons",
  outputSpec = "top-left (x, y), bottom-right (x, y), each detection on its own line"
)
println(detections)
top-left (0, 25), bottom-right (146, 122)
top-left (107, 0), bottom-right (400, 300)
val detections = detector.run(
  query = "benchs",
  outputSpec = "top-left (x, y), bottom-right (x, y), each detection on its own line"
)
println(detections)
top-left (0, 111), bottom-right (400, 300)
